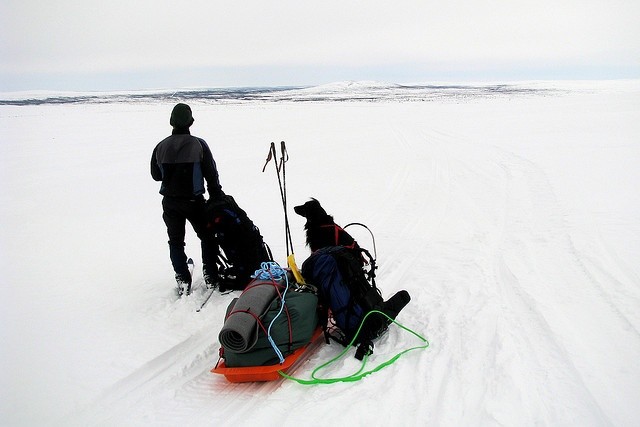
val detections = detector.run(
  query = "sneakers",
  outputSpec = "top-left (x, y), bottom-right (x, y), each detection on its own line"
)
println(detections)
top-left (177, 278), bottom-right (192, 296)
top-left (203, 275), bottom-right (220, 290)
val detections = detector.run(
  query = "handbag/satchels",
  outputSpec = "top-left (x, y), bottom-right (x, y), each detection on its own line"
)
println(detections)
top-left (222, 279), bottom-right (319, 369)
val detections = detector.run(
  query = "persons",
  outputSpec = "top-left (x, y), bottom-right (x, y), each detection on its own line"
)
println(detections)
top-left (150, 103), bottom-right (222, 296)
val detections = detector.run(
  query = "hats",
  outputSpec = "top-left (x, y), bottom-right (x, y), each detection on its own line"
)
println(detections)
top-left (170, 103), bottom-right (194, 128)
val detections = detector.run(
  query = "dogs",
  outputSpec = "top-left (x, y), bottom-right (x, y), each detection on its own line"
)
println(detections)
top-left (293, 197), bottom-right (364, 268)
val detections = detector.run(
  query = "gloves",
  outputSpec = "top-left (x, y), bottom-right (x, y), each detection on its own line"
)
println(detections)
top-left (209, 190), bottom-right (225, 201)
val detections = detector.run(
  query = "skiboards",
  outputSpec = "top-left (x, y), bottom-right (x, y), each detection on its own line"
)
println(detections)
top-left (180, 258), bottom-right (219, 312)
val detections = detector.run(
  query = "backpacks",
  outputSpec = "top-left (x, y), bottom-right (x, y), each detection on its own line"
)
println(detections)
top-left (301, 245), bottom-right (410, 361)
top-left (205, 195), bottom-right (273, 292)
